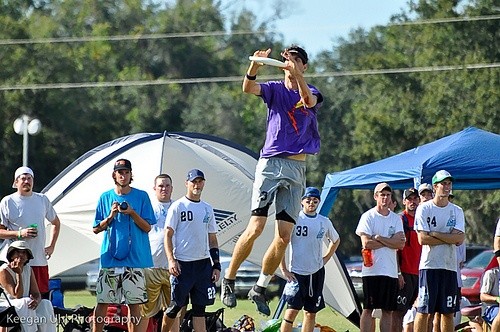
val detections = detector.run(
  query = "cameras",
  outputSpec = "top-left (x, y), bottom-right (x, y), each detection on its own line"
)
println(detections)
top-left (120, 202), bottom-right (128, 210)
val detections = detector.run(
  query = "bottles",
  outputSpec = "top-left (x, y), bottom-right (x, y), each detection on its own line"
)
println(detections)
top-left (359, 248), bottom-right (373, 268)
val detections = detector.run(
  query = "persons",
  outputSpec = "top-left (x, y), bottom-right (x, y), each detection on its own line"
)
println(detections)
top-left (414, 169), bottom-right (465, 332)
top-left (161, 169), bottom-right (220, 332)
top-left (141, 174), bottom-right (182, 332)
top-left (355, 183), bottom-right (406, 332)
top-left (403, 183), bottom-right (433, 332)
top-left (494, 216), bottom-right (500, 267)
top-left (221, 44), bottom-right (324, 316)
top-left (480, 267), bottom-right (500, 332)
top-left (1, 241), bottom-right (56, 332)
top-left (278, 187), bottom-right (340, 332)
top-left (92, 159), bottom-right (157, 332)
top-left (1, 166), bottom-right (60, 299)
top-left (392, 188), bottom-right (422, 332)
top-left (447, 193), bottom-right (466, 332)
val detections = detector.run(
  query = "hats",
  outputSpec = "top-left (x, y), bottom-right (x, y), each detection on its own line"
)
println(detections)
top-left (419, 183), bottom-right (433, 194)
top-left (403, 188), bottom-right (419, 200)
top-left (6, 240), bottom-right (35, 262)
top-left (186, 169), bottom-right (206, 182)
top-left (302, 187), bottom-right (320, 200)
top-left (12, 166), bottom-right (34, 188)
top-left (375, 183), bottom-right (392, 193)
top-left (432, 170), bottom-right (455, 184)
top-left (114, 159), bottom-right (132, 172)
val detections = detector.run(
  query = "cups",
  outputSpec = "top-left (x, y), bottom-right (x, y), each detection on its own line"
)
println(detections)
top-left (27, 224), bottom-right (39, 238)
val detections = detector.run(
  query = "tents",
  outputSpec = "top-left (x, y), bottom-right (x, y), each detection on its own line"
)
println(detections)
top-left (0, 129), bottom-right (363, 330)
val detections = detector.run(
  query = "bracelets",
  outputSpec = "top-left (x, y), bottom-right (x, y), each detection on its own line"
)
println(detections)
top-left (14, 224), bottom-right (26, 240)
top-left (213, 262), bottom-right (221, 271)
top-left (246, 73), bottom-right (256, 80)
top-left (495, 249), bottom-right (500, 257)
top-left (209, 248), bottom-right (219, 261)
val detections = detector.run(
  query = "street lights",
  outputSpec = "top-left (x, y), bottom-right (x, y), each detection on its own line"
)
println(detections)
top-left (13, 113), bottom-right (42, 166)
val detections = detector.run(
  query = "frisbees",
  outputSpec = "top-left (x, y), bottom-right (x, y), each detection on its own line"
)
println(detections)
top-left (249, 56), bottom-right (287, 68)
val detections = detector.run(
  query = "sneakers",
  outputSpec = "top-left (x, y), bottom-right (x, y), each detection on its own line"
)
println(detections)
top-left (221, 277), bottom-right (237, 309)
top-left (247, 286), bottom-right (270, 316)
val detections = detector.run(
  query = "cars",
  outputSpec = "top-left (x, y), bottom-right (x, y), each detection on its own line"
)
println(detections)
top-left (225, 262), bottom-right (281, 296)
top-left (460, 250), bottom-right (499, 305)
